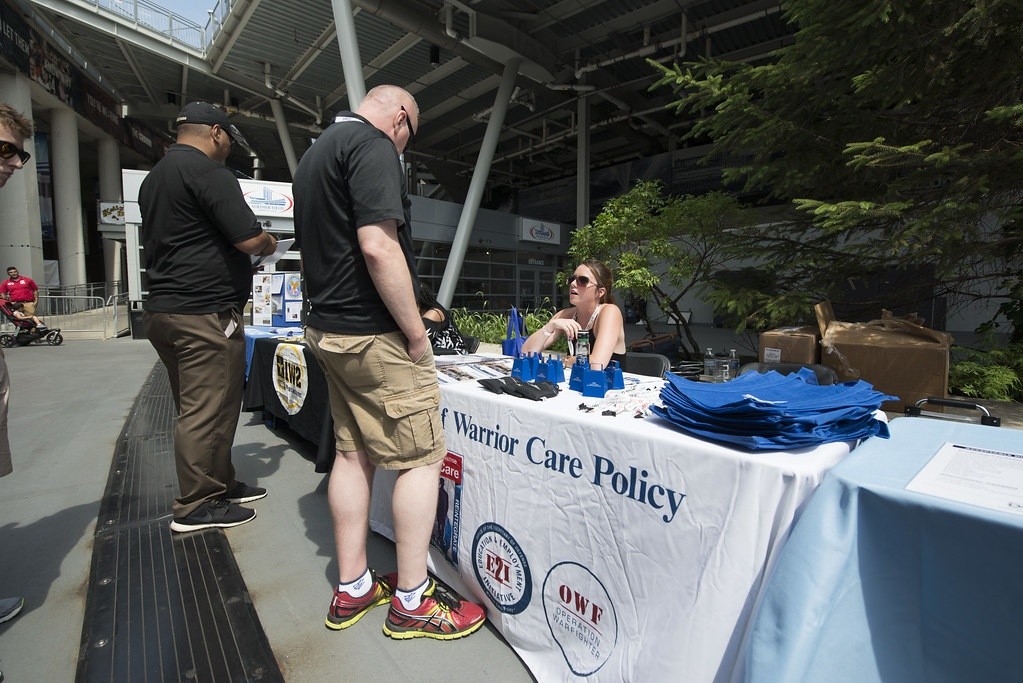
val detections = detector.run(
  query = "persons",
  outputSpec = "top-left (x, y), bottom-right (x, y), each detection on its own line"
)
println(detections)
top-left (0, 104), bottom-right (47, 478)
top-left (519, 258), bottom-right (627, 372)
top-left (137, 102), bottom-right (277, 532)
top-left (292, 83), bottom-right (485, 640)
top-left (414, 283), bottom-right (469, 355)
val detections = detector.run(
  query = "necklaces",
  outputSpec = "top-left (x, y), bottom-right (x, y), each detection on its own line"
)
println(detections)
top-left (572, 304), bottom-right (603, 331)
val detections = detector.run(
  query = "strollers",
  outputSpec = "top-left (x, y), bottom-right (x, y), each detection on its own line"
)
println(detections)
top-left (0, 299), bottom-right (63, 348)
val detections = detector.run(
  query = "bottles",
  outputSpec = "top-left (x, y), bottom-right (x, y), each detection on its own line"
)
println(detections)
top-left (575, 330), bottom-right (589, 364)
top-left (729, 349), bottom-right (740, 377)
top-left (704, 348), bottom-right (716, 376)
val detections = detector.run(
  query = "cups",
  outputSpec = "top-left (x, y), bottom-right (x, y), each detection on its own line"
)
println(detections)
top-left (715, 357), bottom-right (731, 383)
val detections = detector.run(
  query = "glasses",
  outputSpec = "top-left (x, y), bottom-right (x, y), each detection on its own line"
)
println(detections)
top-left (0, 140), bottom-right (31, 165)
top-left (401, 106), bottom-right (415, 153)
top-left (568, 275), bottom-right (601, 288)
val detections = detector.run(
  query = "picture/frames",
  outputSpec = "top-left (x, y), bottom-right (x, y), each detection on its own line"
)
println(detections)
top-left (666, 310), bottom-right (692, 325)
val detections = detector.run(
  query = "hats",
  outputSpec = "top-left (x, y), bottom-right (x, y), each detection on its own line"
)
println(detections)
top-left (176, 101), bottom-right (252, 154)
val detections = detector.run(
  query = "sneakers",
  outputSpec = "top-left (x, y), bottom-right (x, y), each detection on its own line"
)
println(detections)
top-left (381, 578), bottom-right (486, 640)
top-left (224, 481), bottom-right (268, 504)
top-left (325, 571), bottom-right (398, 630)
top-left (170, 497), bottom-right (257, 532)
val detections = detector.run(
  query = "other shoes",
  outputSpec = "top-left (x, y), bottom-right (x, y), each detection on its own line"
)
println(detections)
top-left (0, 597), bottom-right (24, 623)
top-left (36, 323), bottom-right (47, 329)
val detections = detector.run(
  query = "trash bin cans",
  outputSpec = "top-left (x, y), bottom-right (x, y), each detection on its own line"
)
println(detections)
top-left (128, 300), bottom-right (147, 339)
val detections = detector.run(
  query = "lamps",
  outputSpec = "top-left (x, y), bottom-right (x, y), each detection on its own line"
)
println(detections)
top-left (430, 44), bottom-right (440, 67)
top-left (263, 221), bottom-right (272, 227)
top-left (167, 89), bottom-right (176, 106)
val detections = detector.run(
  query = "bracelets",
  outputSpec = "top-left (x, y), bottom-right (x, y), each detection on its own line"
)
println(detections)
top-left (542, 324), bottom-right (555, 336)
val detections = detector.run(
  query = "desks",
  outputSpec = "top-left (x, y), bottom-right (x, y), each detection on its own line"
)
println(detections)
top-left (242, 323), bottom-right (1023, 683)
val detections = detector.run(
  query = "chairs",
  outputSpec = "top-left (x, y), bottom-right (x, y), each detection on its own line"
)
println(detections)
top-left (625, 351), bottom-right (672, 382)
top-left (735, 361), bottom-right (838, 389)
top-left (905, 396), bottom-right (1001, 429)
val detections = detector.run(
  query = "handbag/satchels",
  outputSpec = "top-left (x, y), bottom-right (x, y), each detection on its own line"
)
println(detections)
top-left (476, 306), bottom-right (625, 402)
top-left (649, 367), bottom-right (899, 454)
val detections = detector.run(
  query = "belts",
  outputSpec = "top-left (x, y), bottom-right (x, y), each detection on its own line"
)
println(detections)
top-left (12, 300), bottom-right (34, 303)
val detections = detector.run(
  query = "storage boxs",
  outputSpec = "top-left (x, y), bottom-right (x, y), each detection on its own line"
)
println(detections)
top-left (758, 324), bottom-right (822, 364)
top-left (813, 297), bottom-right (954, 415)
